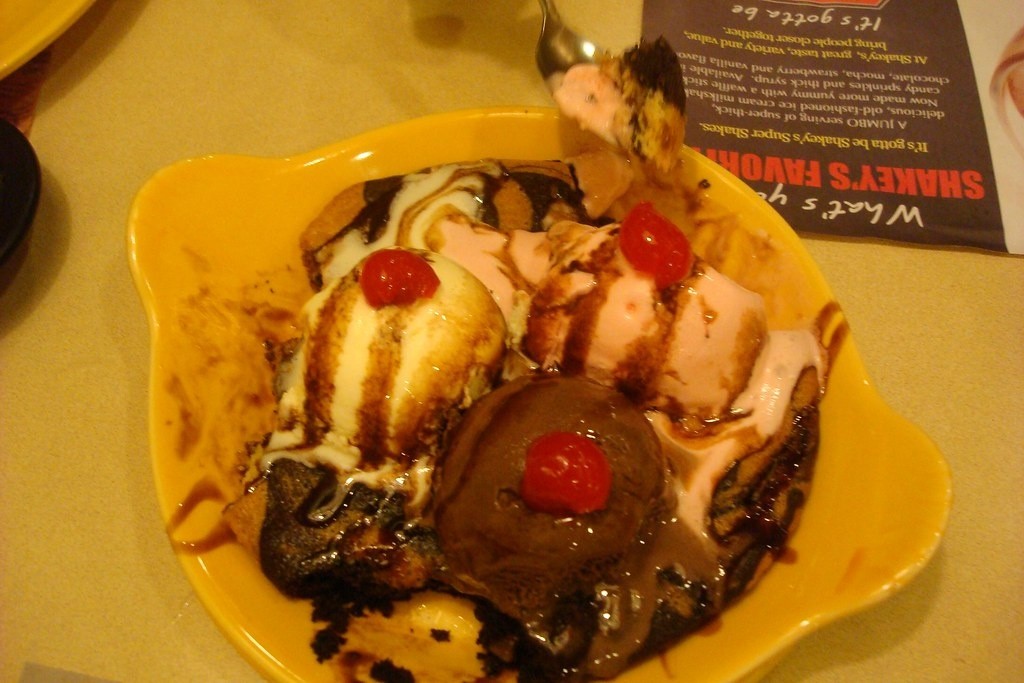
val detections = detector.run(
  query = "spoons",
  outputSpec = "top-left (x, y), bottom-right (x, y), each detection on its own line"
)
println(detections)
top-left (535, 0), bottom-right (604, 96)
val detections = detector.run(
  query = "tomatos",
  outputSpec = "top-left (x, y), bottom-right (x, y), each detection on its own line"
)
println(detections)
top-left (362, 250), bottom-right (439, 305)
top-left (621, 204), bottom-right (687, 283)
top-left (522, 436), bottom-right (611, 511)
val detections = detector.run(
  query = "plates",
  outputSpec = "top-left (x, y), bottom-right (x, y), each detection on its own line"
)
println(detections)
top-left (131, 109), bottom-right (950, 683)
